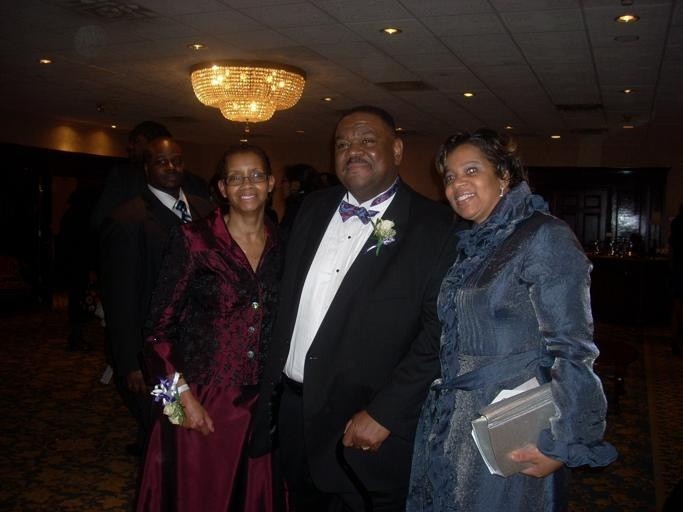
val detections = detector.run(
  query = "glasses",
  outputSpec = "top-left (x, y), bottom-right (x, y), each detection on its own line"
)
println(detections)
top-left (223, 173), bottom-right (267, 186)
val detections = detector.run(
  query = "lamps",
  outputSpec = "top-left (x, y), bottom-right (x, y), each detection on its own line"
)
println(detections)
top-left (188, 61), bottom-right (306, 132)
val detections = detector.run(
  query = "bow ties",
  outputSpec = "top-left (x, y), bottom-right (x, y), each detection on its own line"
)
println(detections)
top-left (340, 200), bottom-right (378, 223)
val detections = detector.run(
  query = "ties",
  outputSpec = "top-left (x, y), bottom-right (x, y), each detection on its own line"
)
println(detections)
top-left (176, 201), bottom-right (192, 223)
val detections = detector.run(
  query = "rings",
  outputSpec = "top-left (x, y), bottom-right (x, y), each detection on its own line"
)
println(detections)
top-left (358, 444), bottom-right (370, 451)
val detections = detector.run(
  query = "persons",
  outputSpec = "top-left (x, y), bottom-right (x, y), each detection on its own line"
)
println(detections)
top-left (277, 163), bottom-right (340, 232)
top-left (667, 201), bottom-right (682, 354)
top-left (268, 104), bottom-right (472, 511)
top-left (138, 141), bottom-right (290, 511)
top-left (90, 120), bottom-right (207, 273)
top-left (404, 124), bottom-right (618, 511)
top-left (99, 135), bottom-right (210, 494)
